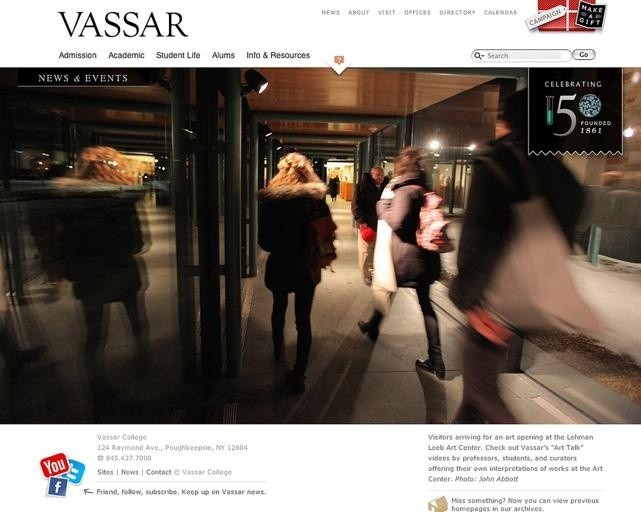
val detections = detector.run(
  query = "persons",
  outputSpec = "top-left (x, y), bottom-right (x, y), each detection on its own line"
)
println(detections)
top-left (256, 151), bottom-right (332, 393)
top-left (327, 173), bottom-right (341, 200)
top-left (26, 146), bottom-right (176, 425)
top-left (445, 86), bottom-right (595, 424)
top-left (359, 146), bottom-right (448, 379)
top-left (351, 166), bottom-right (389, 287)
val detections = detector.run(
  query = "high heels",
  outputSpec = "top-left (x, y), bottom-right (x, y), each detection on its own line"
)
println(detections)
top-left (415, 359), bottom-right (444, 380)
top-left (358, 320), bottom-right (378, 341)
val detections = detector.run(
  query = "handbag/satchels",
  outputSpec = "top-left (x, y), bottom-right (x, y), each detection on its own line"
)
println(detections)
top-left (415, 192), bottom-right (454, 253)
top-left (309, 216), bottom-right (338, 271)
top-left (481, 198), bottom-right (596, 335)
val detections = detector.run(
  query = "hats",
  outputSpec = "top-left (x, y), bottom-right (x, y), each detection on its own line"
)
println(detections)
top-left (359, 224), bottom-right (375, 242)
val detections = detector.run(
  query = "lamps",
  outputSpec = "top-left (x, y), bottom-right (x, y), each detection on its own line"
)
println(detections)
top-left (238, 66), bottom-right (266, 101)
top-left (257, 121), bottom-right (272, 143)
top-left (269, 138), bottom-right (284, 153)
